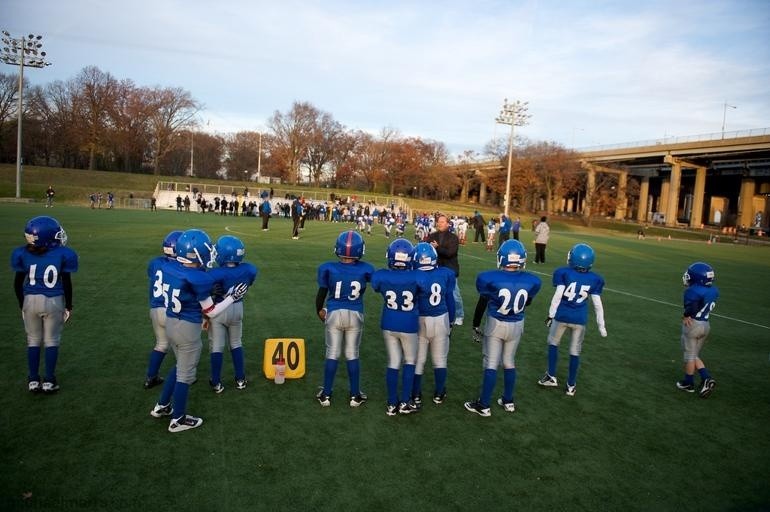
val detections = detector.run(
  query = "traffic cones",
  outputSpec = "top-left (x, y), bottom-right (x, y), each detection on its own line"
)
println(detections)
top-left (657, 237), bottom-right (661, 241)
top-left (707, 239), bottom-right (712, 244)
top-left (667, 233), bottom-right (672, 240)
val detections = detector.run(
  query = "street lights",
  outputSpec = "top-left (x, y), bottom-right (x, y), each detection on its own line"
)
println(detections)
top-left (495, 96), bottom-right (533, 219)
top-left (0, 28), bottom-right (52, 199)
top-left (720, 102), bottom-right (738, 140)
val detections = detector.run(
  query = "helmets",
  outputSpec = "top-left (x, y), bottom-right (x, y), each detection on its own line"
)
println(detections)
top-left (385, 238), bottom-right (415, 268)
top-left (682, 262), bottom-right (715, 286)
top-left (212, 235), bottom-right (246, 264)
top-left (333, 230), bottom-right (366, 259)
top-left (496, 238), bottom-right (528, 270)
top-left (412, 241), bottom-right (439, 269)
top-left (24, 216), bottom-right (68, 248)
top-left (163, 229), bottom-right (217, 268)
top-left (567, 243), bottom-right (595, 270)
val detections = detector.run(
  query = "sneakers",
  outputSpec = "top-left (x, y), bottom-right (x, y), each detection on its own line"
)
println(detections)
top-left (143, 375), bottom-right (164, 390)
top-left (497, 397), bottom-right (515, 412)
top-left (314, 386), bottom-right (331, 408)
top-left (150, 401), bottom-right (174, 418)
top-left (262, 229), bottom-right (269, 231)
top-left (463, 400), bottom-right (491, 418)
top-left (350, 391), bottom-right (368, 407)
top-left (538, 372), bottom-right (560, 387)
top-left (676, 380), bottom-right (695, 393)
top-left (291, 236), bottom-right (299, 240)
top-left (209, 377), bottom-right (225, 394)
top-left (234, 376), bottom-right (247, 389)
top-left (432, 394), bottom-right (447, 404)
top-left (697, 377), bottom-right (717, 399)
top-left (168, 414), bottom-right (205, 433)
top-left (28, 376), bottom-right (60, 394)
top-left (385, 396), bottom-right (424, 416)
top-left (566, 381), bottom-right (577, 396)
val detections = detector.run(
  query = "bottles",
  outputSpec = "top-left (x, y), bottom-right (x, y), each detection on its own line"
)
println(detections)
top-left (275, 361), bottom-right (285, 385)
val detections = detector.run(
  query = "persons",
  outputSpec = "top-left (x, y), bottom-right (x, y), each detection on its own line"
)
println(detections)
top-left (370, 238), bottom-right (419, 416)
top-left (315, 231), bottom-right (375, 408)
top-left (424, 215), bottom-right (466, 324)
top-left (85, 191), bottom-right (114, 211)
top-left (538, 244), bottom-right (608, 396)
top-left (150, 229), bottom-right (212, 433)
top-left (412, 243), bottom-right (456, 408)
top-left (673, 263), bottom-right (720, 398)
top-left (202, 235), bottom-right (259, 394)
top-left (43, 185), bottom-right (56, 209)
top-left (12, 217), bottom-right (77, 396)
top-left (464, 237), bottom-right (544, 416)
top-left (143, 231), bottom-right (185, 389)
top-left (535, 215), bottom-right (549, 264)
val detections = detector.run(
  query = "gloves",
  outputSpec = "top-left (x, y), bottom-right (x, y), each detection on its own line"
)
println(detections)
top-left (63, 309), bottom-right (71, 325)
top-left (544, 317), bottom-right (553, 328)
top-left (472, 327), bottom-right (484, 343)
top-left (231, 281), bottom-right (248, 302)
top-left (449, 321), bottom-right (455, 338)
